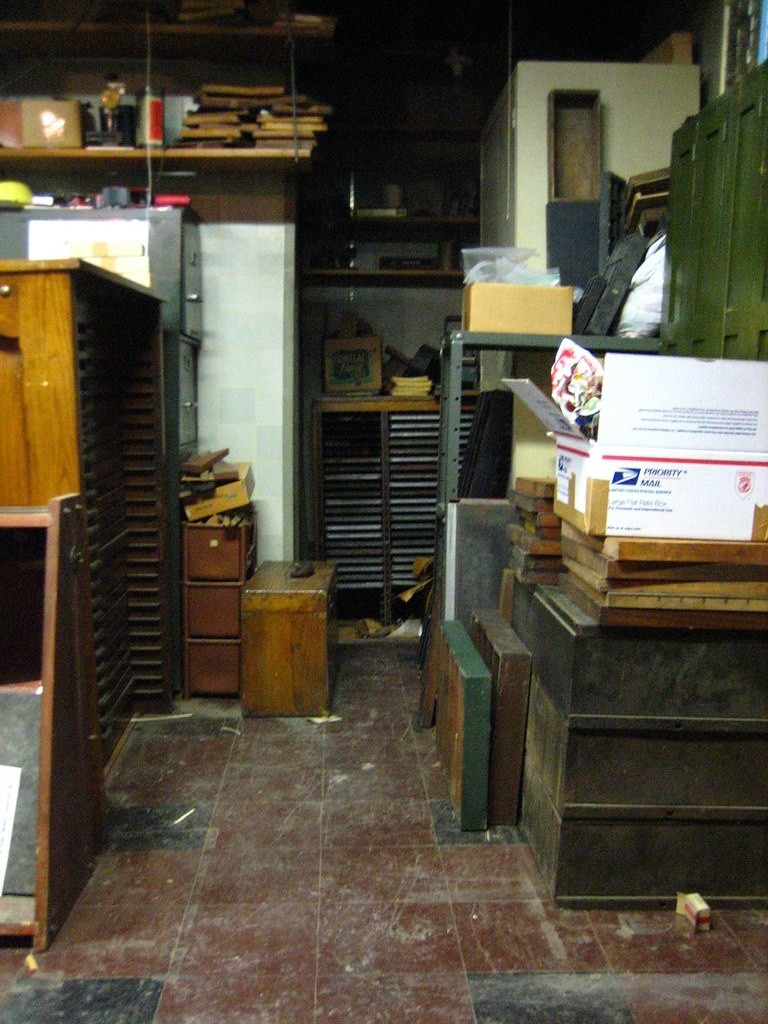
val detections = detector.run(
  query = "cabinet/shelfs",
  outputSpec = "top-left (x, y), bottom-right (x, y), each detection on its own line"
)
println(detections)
top-left (0, 19), bottom-right (334, 172)
top-left (0, 257), bottom-right (176, 770)
top-left (300, 109), bottom-right (479, 282)
top-left (182, 511), bottom-right (258, 702)
top-left (0, 493), bottom-right (104, 952)
top-left (513, 585), bottom-right (768, 911)
top-left (435, 331), bottom-right (667, 619)
top-left (0, 200), bottom-right (203, 459)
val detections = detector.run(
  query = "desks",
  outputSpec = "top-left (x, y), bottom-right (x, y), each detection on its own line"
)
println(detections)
top-left (241, 560), bottom-right (339, 716)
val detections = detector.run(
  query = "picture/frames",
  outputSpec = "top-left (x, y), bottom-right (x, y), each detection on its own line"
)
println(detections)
top-left (326, 335), bottom-right (383, 394)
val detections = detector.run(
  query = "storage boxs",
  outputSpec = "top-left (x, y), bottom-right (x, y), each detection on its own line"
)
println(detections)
top-left (181, 461), bottom-right (255, 521)
top-left (500, 353), bottom-right (768, 542)
top-left (461, 282), bottom-right (574, 335)
top-left (548, 88), bottom-right (600, 202)
